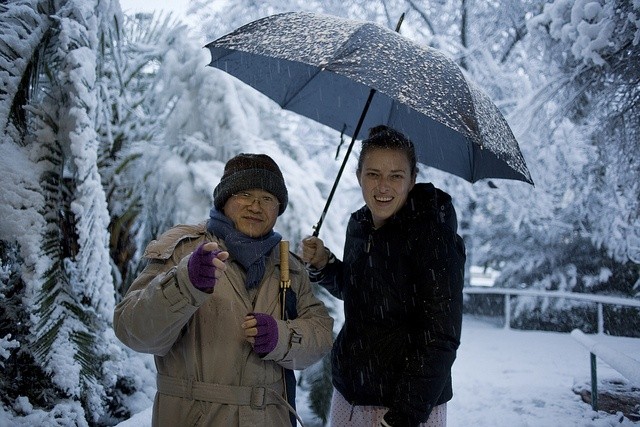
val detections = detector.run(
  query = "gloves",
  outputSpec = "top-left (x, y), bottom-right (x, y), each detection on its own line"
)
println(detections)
top-left (246, 312), bottom-right (279, 355)
top-left (186, 244), bottom-right (221, 293)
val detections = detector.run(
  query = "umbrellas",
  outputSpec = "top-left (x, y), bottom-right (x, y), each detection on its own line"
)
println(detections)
top-left (202, 10), bottom-right (536, 235)
top-left (278, 239), bottom-right (297, 425)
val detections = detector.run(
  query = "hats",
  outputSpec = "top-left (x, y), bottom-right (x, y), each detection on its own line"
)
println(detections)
top-left (213, 153), bottom-right (288, 215)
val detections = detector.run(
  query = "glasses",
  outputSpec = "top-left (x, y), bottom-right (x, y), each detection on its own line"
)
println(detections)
top-left (230, 189), bottom-right (281, 204)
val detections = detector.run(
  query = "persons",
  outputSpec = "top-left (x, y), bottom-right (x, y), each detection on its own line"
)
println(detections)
top-left (303, 124), bottom-right (466, 427)
top-left (114, 153), bottom-right (334, 427)
